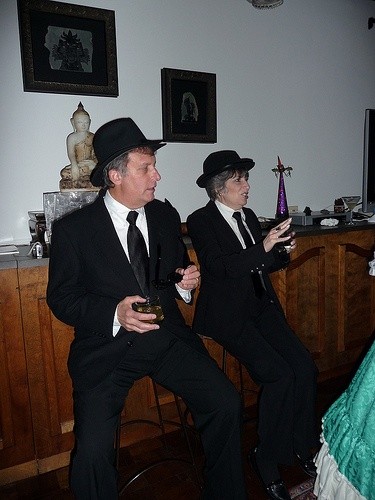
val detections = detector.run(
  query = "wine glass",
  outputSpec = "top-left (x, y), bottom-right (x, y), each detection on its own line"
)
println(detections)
top-left (341, 196), bottom-right (363, 226)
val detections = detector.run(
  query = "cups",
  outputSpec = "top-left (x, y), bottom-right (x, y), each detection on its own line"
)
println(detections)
top-left (276, 228), bottom-right (294, 251)
top-left (132, 295), bottom-right (164, 324)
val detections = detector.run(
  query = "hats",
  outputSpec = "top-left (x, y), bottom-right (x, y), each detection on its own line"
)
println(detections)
top-left (90, 117), bottom-right (166, 187)
top-left (196, 150), bottom-right (254, 188)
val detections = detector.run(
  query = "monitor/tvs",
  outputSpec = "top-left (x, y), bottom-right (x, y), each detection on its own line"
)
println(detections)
top-left (362, 109), bottom-right (375, 213)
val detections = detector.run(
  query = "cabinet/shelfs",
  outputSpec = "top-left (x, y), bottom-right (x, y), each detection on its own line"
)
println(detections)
top-left (0, 230), bottom-right (375, 481)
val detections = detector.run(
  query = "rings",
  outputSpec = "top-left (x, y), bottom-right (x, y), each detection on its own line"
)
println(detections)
top-left (276, 226), bottom-right (281, 231)
top-left (195, 284), bottom-right (199, 289)
top-left (195, 277), bottom-right (199, 283)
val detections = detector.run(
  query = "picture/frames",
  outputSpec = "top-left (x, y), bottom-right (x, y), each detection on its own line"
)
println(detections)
top-left (161, 68), bottom-right (217, 144)
top-left (17, 0), bottom-right (119, 98)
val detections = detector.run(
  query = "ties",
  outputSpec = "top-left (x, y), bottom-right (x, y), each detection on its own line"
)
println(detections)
top-left (232, 212), bottom-right (268, 295)
top-left (127, 211), bottom-right (151, 296)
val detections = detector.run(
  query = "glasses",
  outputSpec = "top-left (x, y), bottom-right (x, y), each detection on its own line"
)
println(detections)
top-left (153, 236), bottom-right (189, 289)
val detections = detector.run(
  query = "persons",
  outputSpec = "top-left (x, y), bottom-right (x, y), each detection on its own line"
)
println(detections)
top-left (46, 117), bottom-right (248, 500)
top-left (187, 150), bottom-right (320, 500)
top-left (60, 101), bottom-right (99, 184)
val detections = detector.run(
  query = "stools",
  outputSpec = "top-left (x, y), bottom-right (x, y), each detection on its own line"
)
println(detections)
top-left (113, 347), bottom-right (257, 500)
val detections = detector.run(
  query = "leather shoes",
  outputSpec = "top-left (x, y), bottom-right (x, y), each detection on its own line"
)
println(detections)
top-left (246, 447), bottom-right (291, 500)
top-left (291, 437), bottom-right (317, 479)
